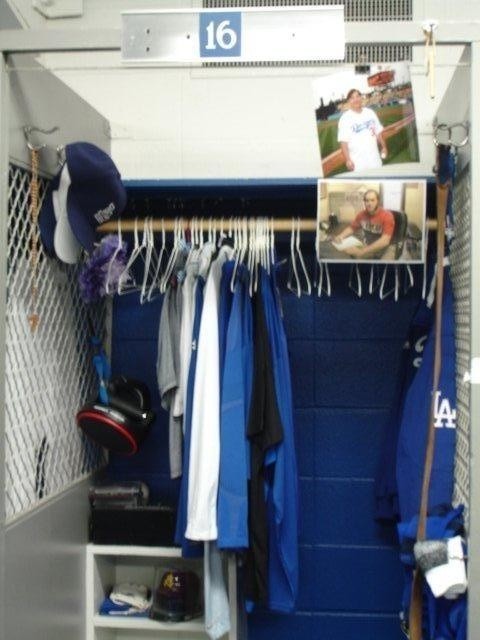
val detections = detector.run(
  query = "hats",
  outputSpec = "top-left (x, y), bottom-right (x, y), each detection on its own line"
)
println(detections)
top-left (41, 141), bottom-right (127, 265)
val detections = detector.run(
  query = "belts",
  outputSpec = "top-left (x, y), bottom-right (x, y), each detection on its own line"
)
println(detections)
top-left (399, 122), bottom-right (455, 640)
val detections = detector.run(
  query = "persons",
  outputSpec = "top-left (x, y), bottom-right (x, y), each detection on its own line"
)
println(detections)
top-left (334, 189), bottom-right (396, 259)
top-left (335, 88), bottom-right (387, 172)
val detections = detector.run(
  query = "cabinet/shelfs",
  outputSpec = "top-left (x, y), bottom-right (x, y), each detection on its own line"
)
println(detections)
top-left (77, 540), bottom-right (240, 640)
